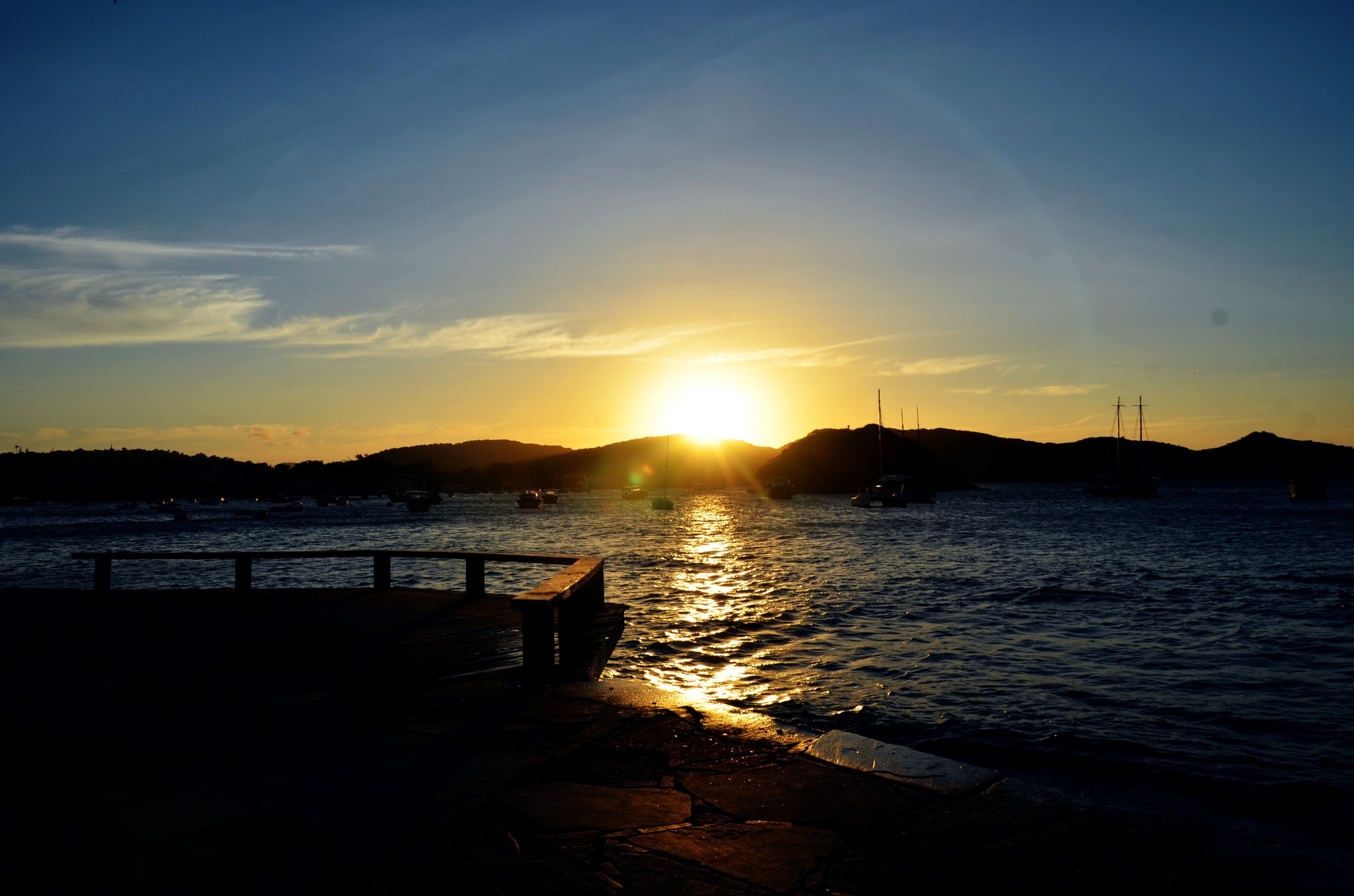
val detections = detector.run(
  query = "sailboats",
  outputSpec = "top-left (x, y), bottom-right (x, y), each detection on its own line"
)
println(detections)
top-left (863, 388), bottom-right (936, 507)
top-left (1090, 394), bottom-right (1167, 497)
top-left (650, 436), bottom-right (675, 510)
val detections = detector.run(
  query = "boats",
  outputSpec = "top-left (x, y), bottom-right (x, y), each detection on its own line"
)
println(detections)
top-left (747, 477), bottom-right (796, 500)
top-left (387, 487), bottom-right (444, 514)
top-left (621, 485), bottom-right (649, 500)
top-left (115, 502), bottom-right (191, 522)
top-left (253, 500), bottom-right (305, 520)
top-left (317, 495), bottom-right (352, 508)
top-left (849, 492), bottom-right (872, 507)
top-left (1290, 480), bottom-right (1327, 501)
top-left (517, 488), bottom-right (560, 509)
